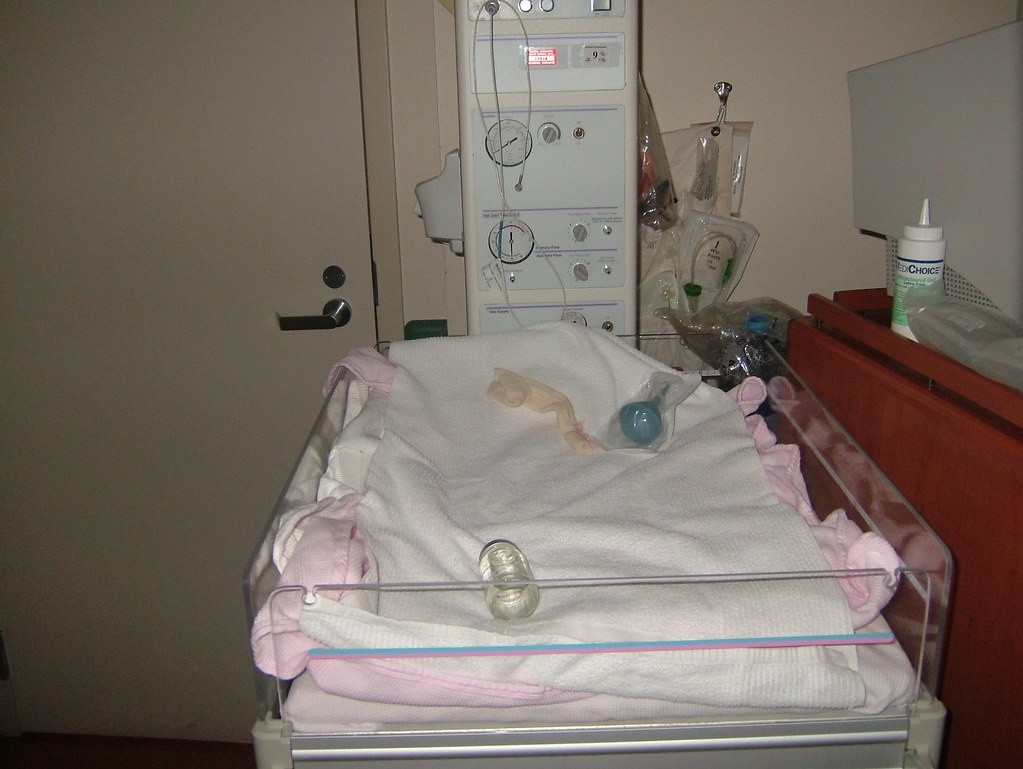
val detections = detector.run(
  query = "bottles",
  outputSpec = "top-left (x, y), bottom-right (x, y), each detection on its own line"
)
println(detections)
top-left (890, 199), bottom-right (948, 341)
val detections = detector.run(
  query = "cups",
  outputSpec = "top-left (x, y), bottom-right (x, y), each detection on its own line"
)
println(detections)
top-left (479, 539), bottom-right (540, 621)
